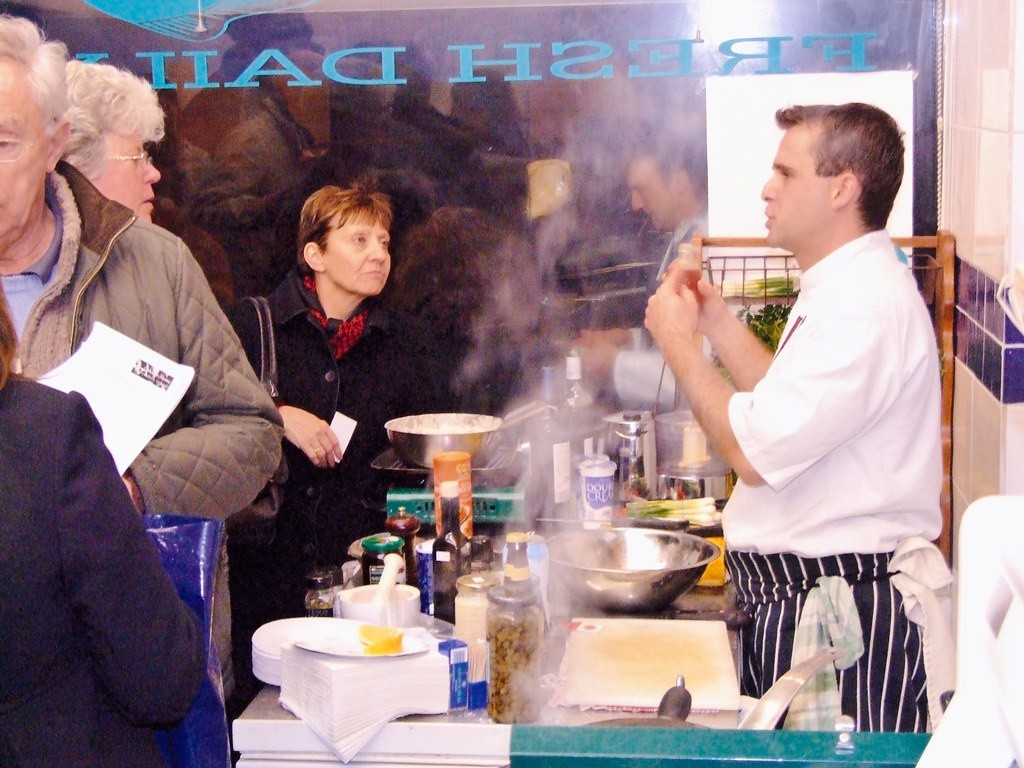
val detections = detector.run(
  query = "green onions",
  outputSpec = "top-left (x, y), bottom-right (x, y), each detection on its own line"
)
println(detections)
top-left (624, 495), bottom-right (723, 527)
top-left (715, 276), bottom-right (800, 295)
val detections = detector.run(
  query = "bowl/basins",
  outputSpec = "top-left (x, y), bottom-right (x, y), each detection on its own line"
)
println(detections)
top-left (546, 526), bottom-right (721, 614)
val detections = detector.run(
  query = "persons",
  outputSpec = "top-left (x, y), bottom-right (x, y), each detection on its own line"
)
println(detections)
top-left (66, 60), bottom-right (164, 226)
top-left (644, 102), bottom-right (942, 736)
top-left (0, 14), bottom-right (286, 703)
top-left (227, 187), bottom-right (536, 710)
top-left (148, 13), bottom-right (543, 341)
top-left (571, 128), bottom-right (705, 410)
top-left (0, 301), bottom-right (207, 768)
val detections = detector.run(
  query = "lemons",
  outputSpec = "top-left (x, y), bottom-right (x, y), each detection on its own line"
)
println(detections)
top-left (358, 623), bottom-right (404, 656)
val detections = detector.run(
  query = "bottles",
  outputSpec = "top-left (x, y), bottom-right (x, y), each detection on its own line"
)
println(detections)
top-left (537, 347), bottom-right (593, 447)
top-left (305, 503), bottom-right (548, 724)
top-left (613, 411), bottom-right (645, 501)
top-left (667, 243), bottom-right (703, 353)
top-left (432, 453), bottom-right (474, 538)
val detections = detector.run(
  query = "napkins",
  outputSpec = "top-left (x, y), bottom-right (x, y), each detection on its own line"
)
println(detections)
top-left (275, 640), bottom-right (450, 763)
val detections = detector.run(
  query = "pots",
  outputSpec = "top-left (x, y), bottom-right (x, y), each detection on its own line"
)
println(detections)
top-left (384, 401), bottom-right (557, 469)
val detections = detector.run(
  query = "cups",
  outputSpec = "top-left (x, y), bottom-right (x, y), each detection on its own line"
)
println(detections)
top-left (578, 460), bottom-right (617, 530)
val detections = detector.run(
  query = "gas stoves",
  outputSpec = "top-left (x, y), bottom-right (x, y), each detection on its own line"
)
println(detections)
top-left (378, 471), bottom-right (551, 533)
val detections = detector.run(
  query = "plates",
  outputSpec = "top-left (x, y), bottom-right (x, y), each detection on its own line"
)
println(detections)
top-left (294, 633), bottom-right (429, 657)
top-left (251, 616), bottom-right (366, 686)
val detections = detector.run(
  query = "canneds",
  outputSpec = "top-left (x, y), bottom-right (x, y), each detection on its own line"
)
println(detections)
top-left (361, 535), bottom-right (406, 585)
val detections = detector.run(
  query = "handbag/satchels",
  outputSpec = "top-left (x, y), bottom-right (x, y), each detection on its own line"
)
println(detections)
top-left (142, 513), bottom-right (232, 768)
top-left (225, 295), bottom-right (289, 548)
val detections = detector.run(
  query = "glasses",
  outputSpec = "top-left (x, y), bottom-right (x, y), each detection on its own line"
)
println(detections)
top-left (108, 151), bottom-right (154, 170)
top-left (0, 117), bottom-right (56, 163)
